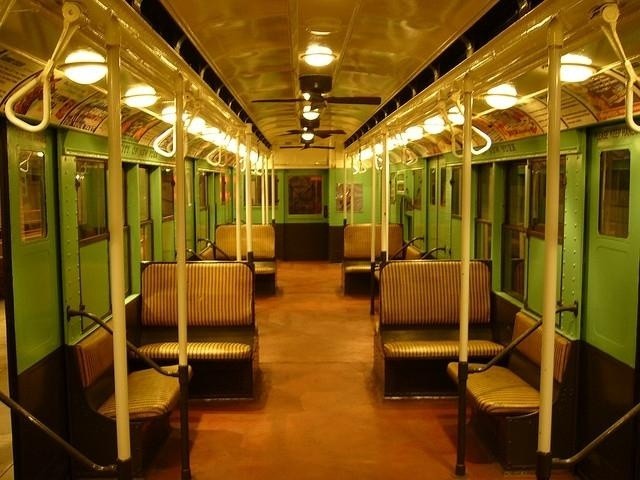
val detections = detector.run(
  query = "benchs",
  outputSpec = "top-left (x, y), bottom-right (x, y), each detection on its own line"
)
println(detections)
top-left (373, 246), bottom-right (427, 282)
top-left (134, 259), bottom-right (259, 404)
top-left (70, 320), bottom-right (194, 473)
top-left (373, 260), bottom-right (506, 401)
top-left (198, 245), bottom-right (217, 261)
top-left (341, 224), bottom-right (404, 297)
top-left (213, 223), bottom-right (277, 297)
top-left (447, 306), bottom-right (578, 471)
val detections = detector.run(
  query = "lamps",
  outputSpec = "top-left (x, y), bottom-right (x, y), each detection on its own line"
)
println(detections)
top-left (299, 130), bottom-right (316, 141)
top-left (58, 45), bottom-right (260, 164)
top-left (297, 45), bottom-right (336, 66)
top-left (300, 105), bottom-right (321, 121)
top-left (350, 52), bottom-right (601, 161)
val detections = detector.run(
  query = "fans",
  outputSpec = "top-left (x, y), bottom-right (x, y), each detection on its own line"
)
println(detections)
top-left (279, 140), bottom-right (338, 152)
top-left (250, 74), bottom-right (381, 109)
top-left (277, 118), bottom-right (346, 138)
